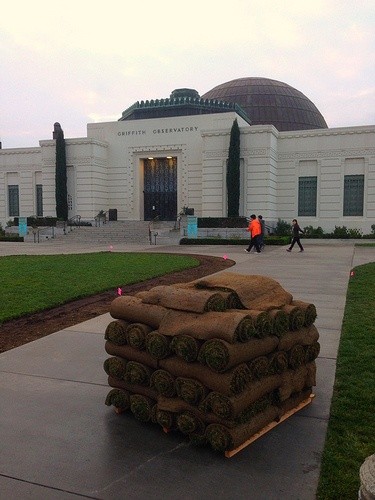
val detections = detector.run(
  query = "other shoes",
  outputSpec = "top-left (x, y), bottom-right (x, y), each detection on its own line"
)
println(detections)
top-left (243, 248), bottom-right (248, 253)
top-left (262, 245), bottom-right (266, 249)
top-left (286, 249), bottom-right (291, 252)
top-left (299, 249), bottom-right (304, 252)
top-left (254, 252), bottom-right (261, 254)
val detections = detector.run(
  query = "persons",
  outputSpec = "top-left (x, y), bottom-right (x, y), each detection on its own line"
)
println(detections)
top-left (253, 215), bottom-right (265, 251)
top-left (244, 214), bottom-right (261, 253)
top-left (287, 219), bottom-right (304, 252)
